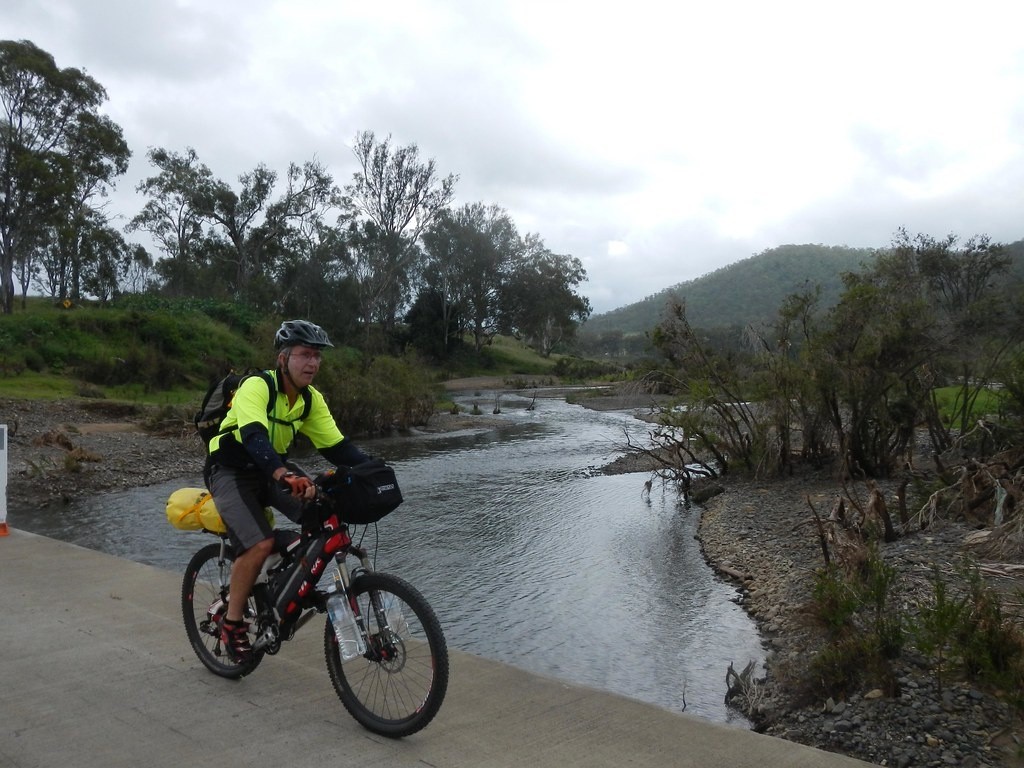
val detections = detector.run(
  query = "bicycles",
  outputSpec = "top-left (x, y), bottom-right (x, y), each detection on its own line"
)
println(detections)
top-left (181, 458), bottom-right (449, 736)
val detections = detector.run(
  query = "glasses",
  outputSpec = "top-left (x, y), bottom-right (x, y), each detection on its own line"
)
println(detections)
top-left (288, 351), bottom-right (321, 364)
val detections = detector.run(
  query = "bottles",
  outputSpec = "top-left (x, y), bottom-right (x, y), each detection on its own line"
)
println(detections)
top-left (379, 591), bottom-right (412, 644)
top-left (326, 585), bottom-right (367, 661)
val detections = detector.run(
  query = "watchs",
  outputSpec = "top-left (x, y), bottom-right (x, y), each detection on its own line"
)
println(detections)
top-left (280, 469), bottom-right (298, 484)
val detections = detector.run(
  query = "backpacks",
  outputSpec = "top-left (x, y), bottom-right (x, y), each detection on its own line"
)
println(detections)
top-left (194, 367), bottom-right (312, 443)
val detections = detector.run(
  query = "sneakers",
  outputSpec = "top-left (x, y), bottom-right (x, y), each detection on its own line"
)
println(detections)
top-left (302, 586), bottom-right (331, 614)
top-left (218, 612), bottom-right (255, 666)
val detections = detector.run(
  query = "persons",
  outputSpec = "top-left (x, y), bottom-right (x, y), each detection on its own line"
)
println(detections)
top-left (202, 320), bottom-right (379, 667)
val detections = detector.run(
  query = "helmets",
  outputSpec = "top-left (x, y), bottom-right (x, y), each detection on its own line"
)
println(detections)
top-left (272, 320), bottom-right (335, 349)
